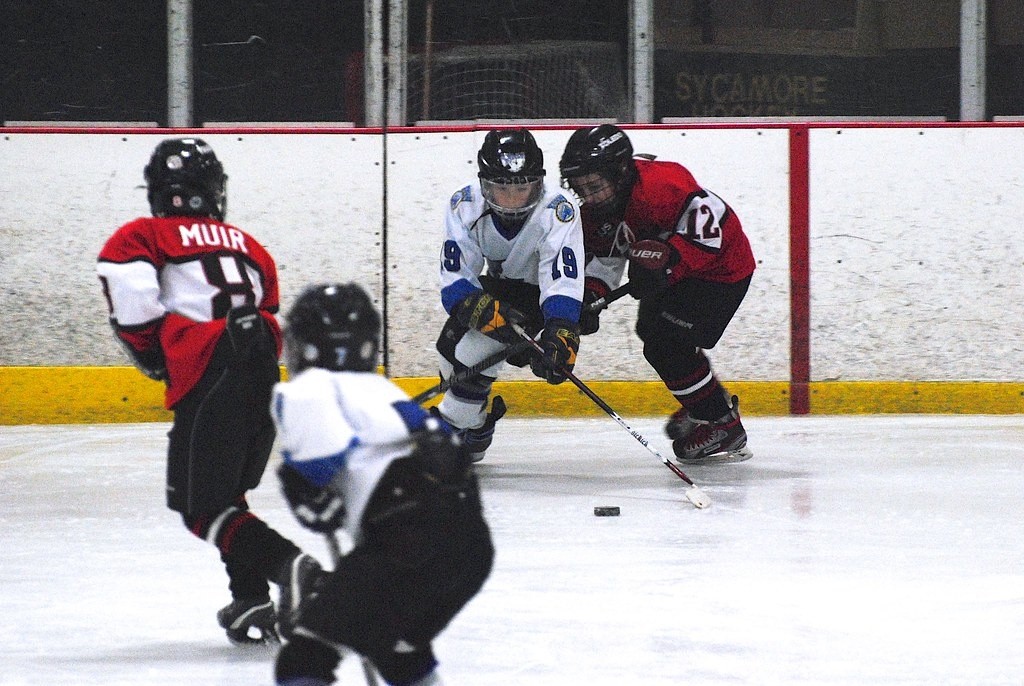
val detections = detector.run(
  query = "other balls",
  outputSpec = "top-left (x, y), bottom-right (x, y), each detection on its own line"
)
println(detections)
top-left (594, 506), bottom-right (620, 517)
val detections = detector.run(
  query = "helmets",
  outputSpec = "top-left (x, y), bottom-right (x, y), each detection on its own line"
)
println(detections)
top-left (144, 138), bottom-right (228, 223)
top-left (559, 124), bottom-right (634, 222)
top-left (285, 283), bottom-right (381, 373)
top-left (477, 127), bottom-right (546, 222)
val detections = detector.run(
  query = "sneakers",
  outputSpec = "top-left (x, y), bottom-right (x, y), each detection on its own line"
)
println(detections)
top-left (421, 395), bottom-right (507, 463)
top-left (673, 395), bottom-right (753, 464)
top-left (267, 541), bottom-right (322, 637)
top-left (665, 406), bottom-right (716, 440)
top-left (216, 547), bottom-right (275, 643)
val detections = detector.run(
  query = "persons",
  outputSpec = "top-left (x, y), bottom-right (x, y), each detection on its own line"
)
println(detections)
top-left (559, 125), bottom-right (757, 460)
top-left (94, 137), bottom-right (321, 639)
top-left (432, 128), bottom-right (585, 464)
top-left (269, 280), bottom-right (497, 686)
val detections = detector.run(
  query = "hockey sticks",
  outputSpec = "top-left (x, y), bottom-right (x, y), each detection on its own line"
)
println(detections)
top-left (325, 531), bottom-right (378, 686)
top-left (413, 282), bottom-right (629, 406)
top-left (509, 319), bottom-right (712, 509)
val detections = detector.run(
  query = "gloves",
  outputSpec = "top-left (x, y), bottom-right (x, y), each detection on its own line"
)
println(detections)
top-left (624, 237), bottom-right (681, 300)
top-left (450, 290), bottom-right (527, 345)
top-left (574, 276), bottom-right (611, 335)
top-left (529, 318), bottom-right (581, 385)
